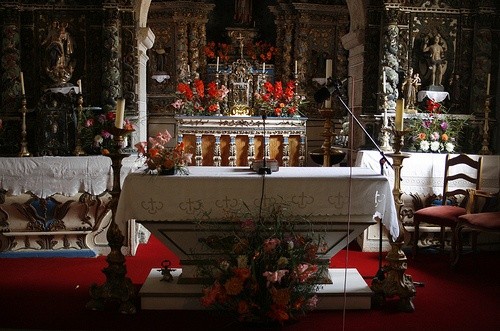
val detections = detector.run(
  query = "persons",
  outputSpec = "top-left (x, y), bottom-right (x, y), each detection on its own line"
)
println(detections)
top-left (423, 35), bottom-right (442, 86)
top-left (403, 67), bottom-right (418, 110)
top-left (42, 20), bottom-right (72, 55)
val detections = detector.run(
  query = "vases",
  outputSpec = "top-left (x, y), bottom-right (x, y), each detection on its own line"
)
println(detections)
top-left (159, 164), bottom-right (177, 176)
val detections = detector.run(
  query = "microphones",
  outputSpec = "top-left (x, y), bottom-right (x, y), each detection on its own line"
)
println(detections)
top-left (257, 114), bottom-right (272, 174)
top-left (314, 76), bottom-right (349, 104)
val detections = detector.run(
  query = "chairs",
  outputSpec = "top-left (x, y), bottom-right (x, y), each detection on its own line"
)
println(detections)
top-left (453, 210), bottom-right (500, 271)
top-left (409, 151), bottom-right (486, 274)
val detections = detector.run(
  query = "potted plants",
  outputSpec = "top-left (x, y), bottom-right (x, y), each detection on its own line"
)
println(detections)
top-left (216, 56), bottom-right (220, 71)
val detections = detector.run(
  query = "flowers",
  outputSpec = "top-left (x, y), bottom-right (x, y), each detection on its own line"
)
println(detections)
top-left (252, 79), bottom-right (307, 115)
top-left (67, 110), bottom-right (148, 152)
top-left (387, 98), bottom-right (475, 153)
top-left (135, 126), bottom-right (187, 175)
top-left (171, 80), bottom-right (232, 113)
top-left (187, 191), bottom-right (324, 324)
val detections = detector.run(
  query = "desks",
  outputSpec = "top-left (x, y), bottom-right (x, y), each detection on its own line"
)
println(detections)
top-left (111, 163), bottom-right (400, 287)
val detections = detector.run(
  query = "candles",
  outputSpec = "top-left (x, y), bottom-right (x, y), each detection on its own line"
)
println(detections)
top-left (78, 79), bottom-right (82, 94)
top-left (294, 59), bottom-right (297, 74)
top-left (262, 62), bottom-right (266, 73)
top-left (115, 95), bottom-right (126, 128)
top-left (393, 96), bottom-right (406, 129)
top-left (20, 70), bottom-right (27, 96)
top-left (186, 64), bottom-right (190, 72)
